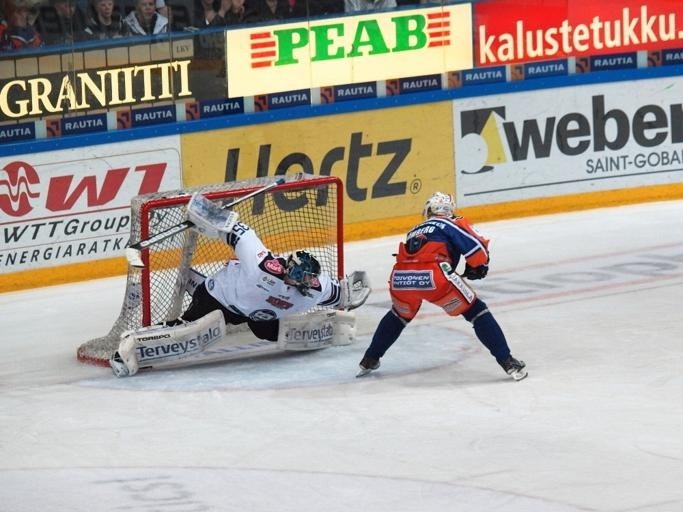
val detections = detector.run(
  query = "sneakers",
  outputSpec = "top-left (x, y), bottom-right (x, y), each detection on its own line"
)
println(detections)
top-left (499, 355), bottom-right (525, 373)
top-left (360, 355), bottom-right (380, 370)
top-left (110, 350), bottom-right (129, 375)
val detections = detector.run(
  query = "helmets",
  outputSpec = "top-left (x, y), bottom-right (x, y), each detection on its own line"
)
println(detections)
top-left (424, 192), bottom-right (456, 219)
top-left (284, 250), bottom-right (320, 291)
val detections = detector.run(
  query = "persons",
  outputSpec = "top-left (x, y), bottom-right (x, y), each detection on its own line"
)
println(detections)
top-left (109, 190), bottom-right (375, 379)
top-left (355, 191), bottom-right (527, 376)
top-left (2, 2), bottom-right (423, 52)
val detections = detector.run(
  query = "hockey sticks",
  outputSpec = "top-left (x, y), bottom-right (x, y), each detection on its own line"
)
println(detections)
top-left (125, 179), bottom-right (284, 268)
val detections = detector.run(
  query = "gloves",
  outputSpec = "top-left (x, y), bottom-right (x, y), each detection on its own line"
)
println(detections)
top-left (463, 263), bottom-right (488, 280)
